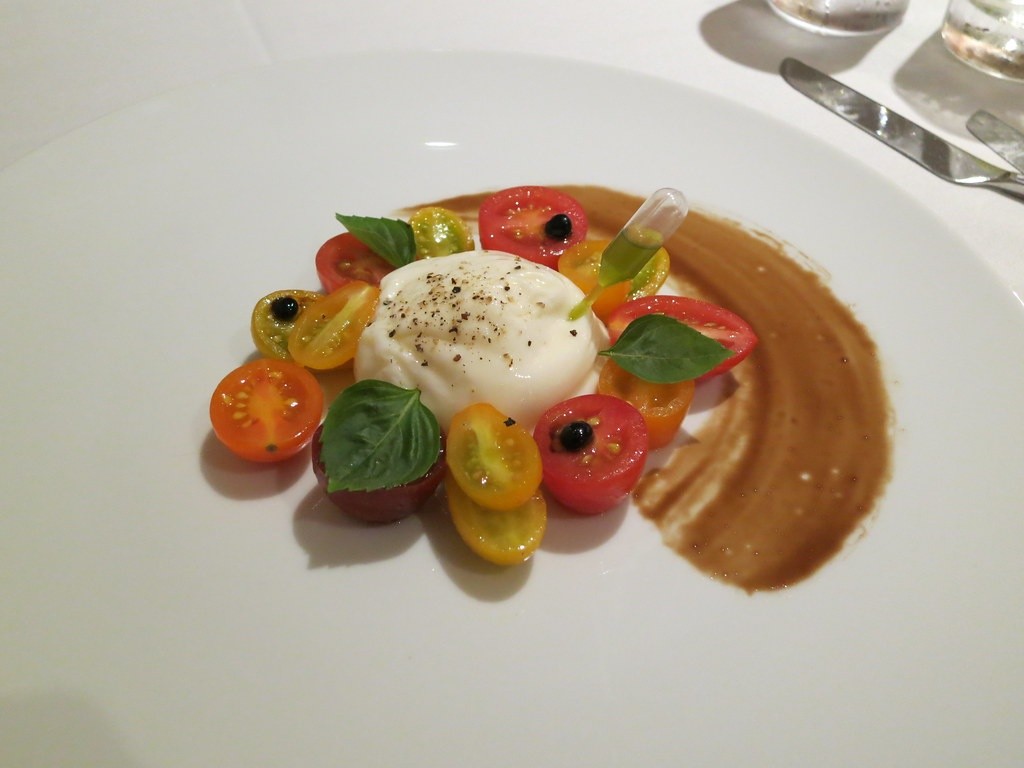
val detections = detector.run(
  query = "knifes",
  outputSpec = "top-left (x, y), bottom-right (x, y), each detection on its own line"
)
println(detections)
top-left (780, 57), bottom-right (1024, 199)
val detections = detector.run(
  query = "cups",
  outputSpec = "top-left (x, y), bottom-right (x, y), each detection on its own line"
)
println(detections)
top-left (765, 0), bottom-right (911, 37)
top-left (940, 0), bottom-right (1024, 82)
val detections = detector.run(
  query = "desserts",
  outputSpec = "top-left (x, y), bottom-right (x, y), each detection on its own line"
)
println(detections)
top-left (354, 248), bottom-right (607, 441)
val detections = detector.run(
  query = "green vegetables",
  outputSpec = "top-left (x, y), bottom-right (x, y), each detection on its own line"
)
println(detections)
top-left (318, 371), bottom-right (439, 492)
top-left (335, 213), bottom-right (417, 268)
top-left (600, 313), bottom-right (738, 380)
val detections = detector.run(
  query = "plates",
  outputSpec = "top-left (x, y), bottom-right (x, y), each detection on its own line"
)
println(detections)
top-left (0, 49), bottom-right (1024, 768)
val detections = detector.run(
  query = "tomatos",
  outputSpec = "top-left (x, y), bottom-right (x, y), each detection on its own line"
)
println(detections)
top-left (208, 187), bottom-right (758, 566)
